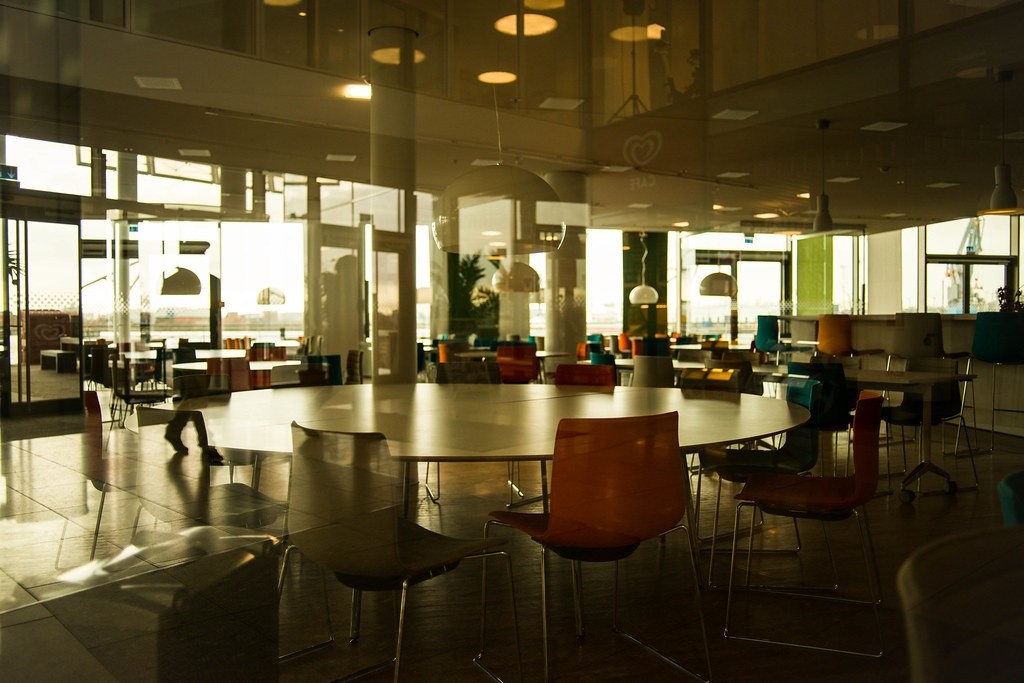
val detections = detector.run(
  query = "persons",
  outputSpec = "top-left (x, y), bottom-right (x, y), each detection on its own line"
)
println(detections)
top-left (164, 371), bottom-right (225, 466)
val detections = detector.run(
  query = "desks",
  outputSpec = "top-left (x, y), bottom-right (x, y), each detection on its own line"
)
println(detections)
top-left (61, 342), bottom-right (978, 645)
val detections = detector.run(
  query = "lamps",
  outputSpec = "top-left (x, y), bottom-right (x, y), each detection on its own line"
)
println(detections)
top-left (493, 260), bottom-right (540, 292)
top-left (700, 249), bottom-right (739, 296)
top-left (629, 232), bottom-right (659, 304)
top-left (813, 117), bottom-right (834, 233)
top-left (160, 265), bottom-right (202, 295)
top-left (430, 165), bottom-right (566, 256)
top-left (990, 70), bottom-right (1018, 211)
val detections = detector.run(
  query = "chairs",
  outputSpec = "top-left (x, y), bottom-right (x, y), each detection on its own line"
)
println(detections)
top-left (39, 312), bottom-right (1024, 683)
